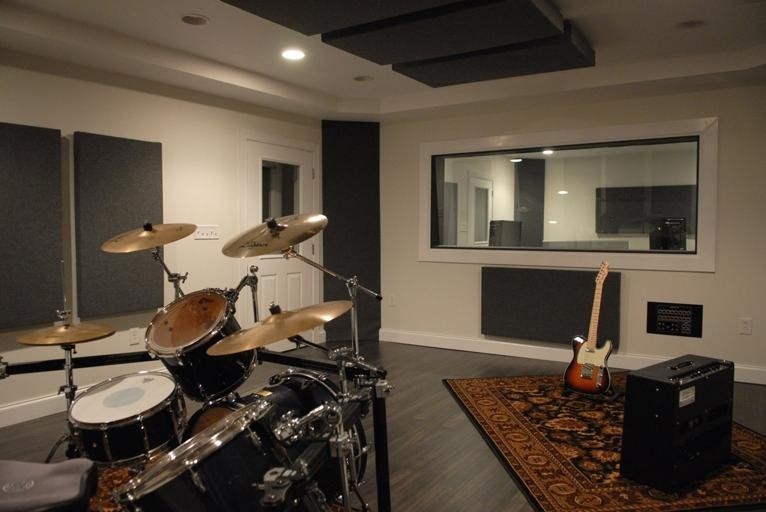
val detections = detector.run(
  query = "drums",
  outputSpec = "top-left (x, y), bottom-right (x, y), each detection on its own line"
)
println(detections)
top-left (67, 372), bottom-right (187, 467)
top-left (144, 288), bottom-right (257, 402)
top-left (118, 401), bottom-right (329, 511)
top-left (181, 370), bottom-right (369, 507)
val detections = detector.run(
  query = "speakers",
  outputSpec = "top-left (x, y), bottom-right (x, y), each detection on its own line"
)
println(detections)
top-left (619, 353), bottom-right (735, 496)
top-left (649, 217), bottom-right (687, 251)
top-left (489, 220), bottom-right (521, 248)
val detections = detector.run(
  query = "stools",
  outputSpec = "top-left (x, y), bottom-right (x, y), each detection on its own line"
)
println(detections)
top-left (1, 457), bottom-right (99, 511)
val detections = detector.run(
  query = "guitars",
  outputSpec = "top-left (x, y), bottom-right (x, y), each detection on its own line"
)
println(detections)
top-left (563, 261), bottom-right (613, 397)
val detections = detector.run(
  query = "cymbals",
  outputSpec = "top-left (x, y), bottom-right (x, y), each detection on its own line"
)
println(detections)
top-left (206, 300), bottom-right (353, 355)
top-left (100, 224), bottom-right (197, 253)
top-left (222, 213), bottom-right (328, 258)
top-left (16, 323), bottom-right (116, 345)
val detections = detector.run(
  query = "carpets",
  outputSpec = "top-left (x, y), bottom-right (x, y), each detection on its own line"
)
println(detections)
top-left (443, 369), bottom-right (766, 510)
